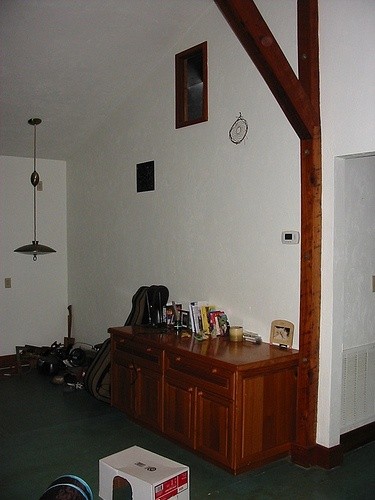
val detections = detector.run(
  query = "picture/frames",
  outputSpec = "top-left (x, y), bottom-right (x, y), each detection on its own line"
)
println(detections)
top-left (269, 319), bottom-right (294, 349)
top-left (174, 40), bottom-right (209, 129)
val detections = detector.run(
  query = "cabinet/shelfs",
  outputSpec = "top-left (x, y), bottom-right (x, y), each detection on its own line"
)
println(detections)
top-left (162, 328), bottom-right (301, 478)
top-left (107, 325), bottom-right (179, 435)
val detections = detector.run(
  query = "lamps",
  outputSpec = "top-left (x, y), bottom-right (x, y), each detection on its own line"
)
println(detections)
top-left (13, 118), bottom-right (57, 261)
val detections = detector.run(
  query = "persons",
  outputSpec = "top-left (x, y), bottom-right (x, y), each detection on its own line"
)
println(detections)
top-left (275, 328), bottom-right (290, 340)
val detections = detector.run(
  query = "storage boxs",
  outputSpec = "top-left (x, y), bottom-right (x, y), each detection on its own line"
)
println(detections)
top-left (99, 445), bottom-right (190, 500)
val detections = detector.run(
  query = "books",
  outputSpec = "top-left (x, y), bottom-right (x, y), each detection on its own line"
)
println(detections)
top-left (156, 301), bottom-right (230, 336)
